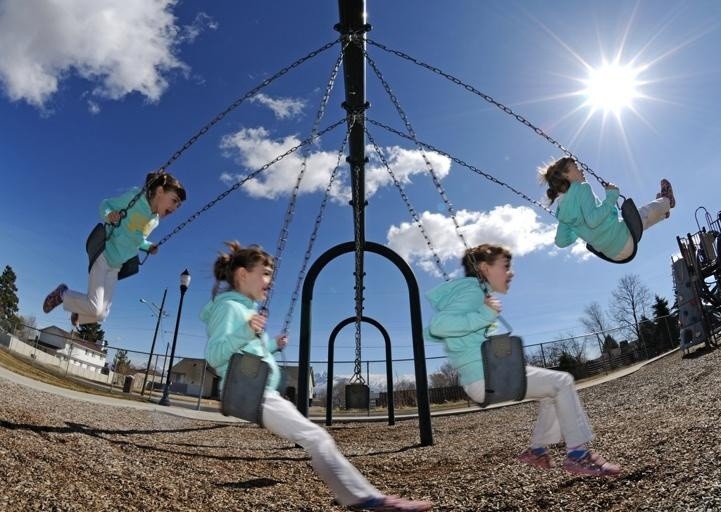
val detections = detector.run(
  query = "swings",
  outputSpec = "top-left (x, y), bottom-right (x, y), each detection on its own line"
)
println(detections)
top-left (85, 30), bottom-right (645, 429)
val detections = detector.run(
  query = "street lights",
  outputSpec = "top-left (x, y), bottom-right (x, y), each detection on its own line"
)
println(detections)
top-left (156, 268), bottom-right (192, 407)
top-left (137, 287), bottom-right (168, 396)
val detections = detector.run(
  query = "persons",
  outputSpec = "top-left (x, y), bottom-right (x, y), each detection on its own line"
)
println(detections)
top-left (423, 243), bottom-right (621, 477)
top-left (199, 240), bottom-right (435, 512)
top-left (537, 155), bottom-right (676, 262)
top-left (42, 171), bottom-right (187, 326)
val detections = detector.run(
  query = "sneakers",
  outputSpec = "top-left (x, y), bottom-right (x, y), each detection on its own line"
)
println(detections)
top-left (564, 449), bottom-right (619, 474)
top-left (657, 179), bottom-right (675, 219)
top-left (519, 446), bottom-right (555, 468)
top-left (43, 284), bottom-right (80, 328)
